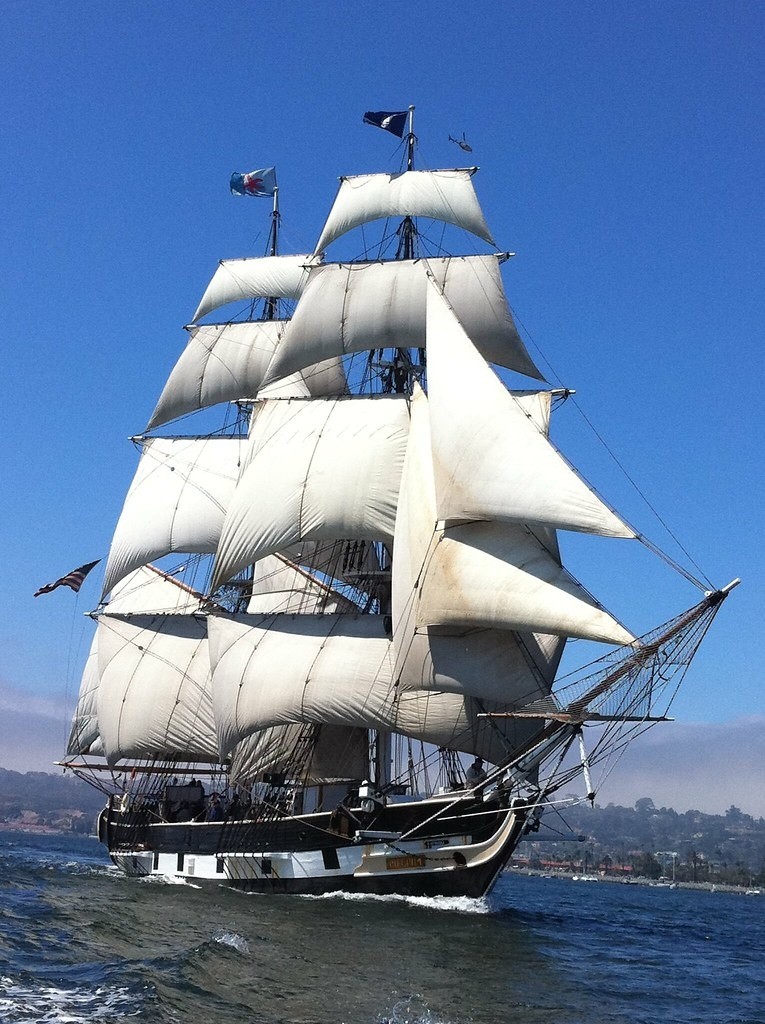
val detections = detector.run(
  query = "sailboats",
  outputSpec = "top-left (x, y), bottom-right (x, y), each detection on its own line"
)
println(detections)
top-left (32, 105), bottom-right (740, 912)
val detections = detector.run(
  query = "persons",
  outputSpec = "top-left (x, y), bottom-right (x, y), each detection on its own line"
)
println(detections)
top-left (169, 775), bottom-right (287, 823)
top-left (466, 757), bottom-right (488, 795)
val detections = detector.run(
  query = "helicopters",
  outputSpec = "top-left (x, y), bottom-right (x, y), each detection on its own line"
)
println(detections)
top-left (449, 131), bottom-right (472, 152)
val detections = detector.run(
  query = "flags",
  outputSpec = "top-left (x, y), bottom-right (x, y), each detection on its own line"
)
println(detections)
top-left (363, 111), bottom-right (409, 138)
top-left (230, 166), bottom-right (277, 198)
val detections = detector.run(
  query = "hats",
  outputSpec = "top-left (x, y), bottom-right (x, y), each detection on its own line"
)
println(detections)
top-left (475, 757), bottom-right (485, 763)
